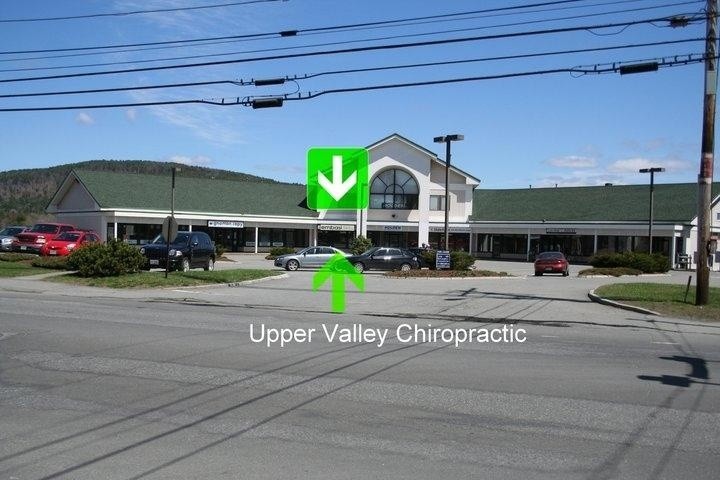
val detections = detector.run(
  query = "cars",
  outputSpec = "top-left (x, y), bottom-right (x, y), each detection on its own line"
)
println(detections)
top-left (275, 245), bottom-right (353, 272)
top-left (341, 246), bottom-right (422, 273)
top-left (533, 251), bottom-right (570, 277)
top-left (1, 222), bottom-right (101, 257)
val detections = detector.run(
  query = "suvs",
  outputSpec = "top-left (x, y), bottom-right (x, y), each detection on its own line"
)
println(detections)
top-left (140, 230), bottom-right (216, 271)
